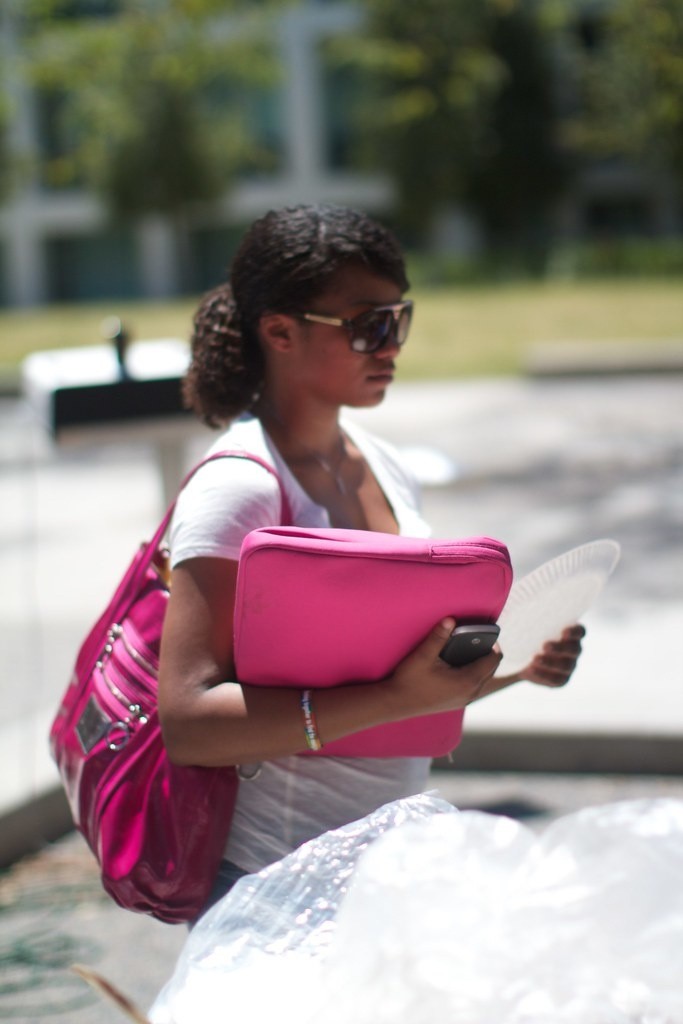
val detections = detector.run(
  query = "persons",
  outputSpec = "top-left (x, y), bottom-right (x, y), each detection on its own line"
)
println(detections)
top-left (156, 202), bottom-right (587, 935)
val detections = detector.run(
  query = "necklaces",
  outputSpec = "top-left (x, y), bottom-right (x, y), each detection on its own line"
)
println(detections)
top-left (258, 398), bottom-right (348, 496)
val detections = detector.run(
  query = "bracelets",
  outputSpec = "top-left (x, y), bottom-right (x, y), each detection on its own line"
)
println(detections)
top-left (300, 689), bottom-right (323, 751)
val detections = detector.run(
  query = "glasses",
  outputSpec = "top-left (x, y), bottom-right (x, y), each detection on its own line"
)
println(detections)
top-left (282, 300), bottom-right (413, 354)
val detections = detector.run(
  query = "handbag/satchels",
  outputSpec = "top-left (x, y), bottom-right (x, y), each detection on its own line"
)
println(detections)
top-left (48, 452), bottom-right (293, 924)
top-left (232, 524), bottom-right (513, 759)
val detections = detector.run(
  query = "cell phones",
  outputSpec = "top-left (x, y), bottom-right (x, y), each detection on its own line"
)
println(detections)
top-left (441, 625), bottom-right (500, 668)
